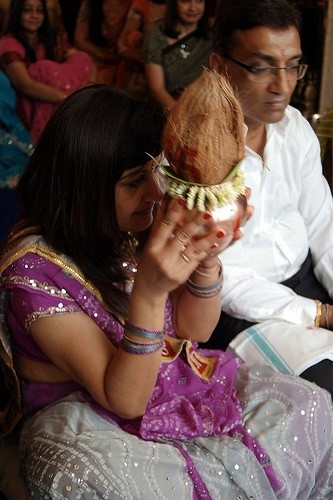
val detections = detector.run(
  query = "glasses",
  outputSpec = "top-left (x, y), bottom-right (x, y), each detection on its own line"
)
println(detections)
top-left (219, 53), bottom-right (308, 79)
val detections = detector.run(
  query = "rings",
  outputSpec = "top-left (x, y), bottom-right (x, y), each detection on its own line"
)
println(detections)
top-left (180, 253), bottom-right (192, 264)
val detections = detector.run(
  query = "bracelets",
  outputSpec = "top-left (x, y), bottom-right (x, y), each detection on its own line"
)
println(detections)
top-left (325, 303), bottom-right (329, 329)
top-left (185, 257), bottom-right (224, 299)
top-left (122, 322), bottom-right (165, 354)
top-left (315, 300), bottom-right (322, 327)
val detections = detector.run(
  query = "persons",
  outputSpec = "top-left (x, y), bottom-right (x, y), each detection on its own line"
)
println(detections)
top-left (144, 0), bottom-right (226, 112)
top-left (73, 0), bottom-right (142, 90)
top-left (0, 0), bottom-right (96, 140)
top-left (154, 0), bottom-right (333, 407)
top-left (0, 84), bottom-right (333, 500)
top-left (117, 0), bottom-right (173, 101)
